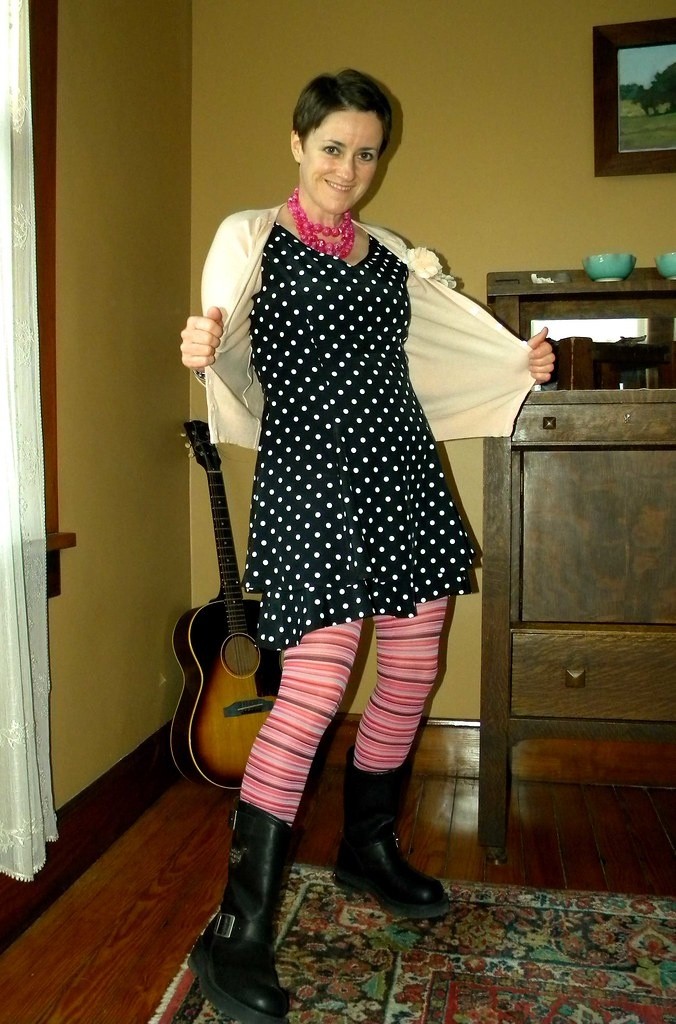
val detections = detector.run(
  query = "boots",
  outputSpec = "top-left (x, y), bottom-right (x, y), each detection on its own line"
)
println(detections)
top-left (337, 746), bottom-right (449, 921)
top-left (188, 799), bottom-right (303, 1023)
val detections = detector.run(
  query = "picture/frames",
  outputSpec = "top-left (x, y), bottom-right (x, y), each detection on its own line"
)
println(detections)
top-left (591, 16), bottom-right (676, 179)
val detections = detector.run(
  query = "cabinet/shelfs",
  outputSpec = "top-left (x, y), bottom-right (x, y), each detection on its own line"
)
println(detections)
top-left (474, 255), bottom-right (676, 875)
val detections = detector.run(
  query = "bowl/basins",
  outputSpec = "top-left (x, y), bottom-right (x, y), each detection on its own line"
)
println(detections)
top-left (582, 253), bottom-right (637, 283)
top-left (654, 252), bottom-right (676, 281)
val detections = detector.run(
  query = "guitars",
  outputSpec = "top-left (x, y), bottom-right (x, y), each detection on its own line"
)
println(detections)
top-left (171, 419), bottom-right (324, 792)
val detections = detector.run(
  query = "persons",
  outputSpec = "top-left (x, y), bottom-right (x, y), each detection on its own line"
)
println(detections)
top-left (180, 67), bottom-right (557, 1024)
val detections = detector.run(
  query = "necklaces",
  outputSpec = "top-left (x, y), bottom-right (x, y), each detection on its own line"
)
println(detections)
top-left (286, 187), bottom-right (356, 260)
